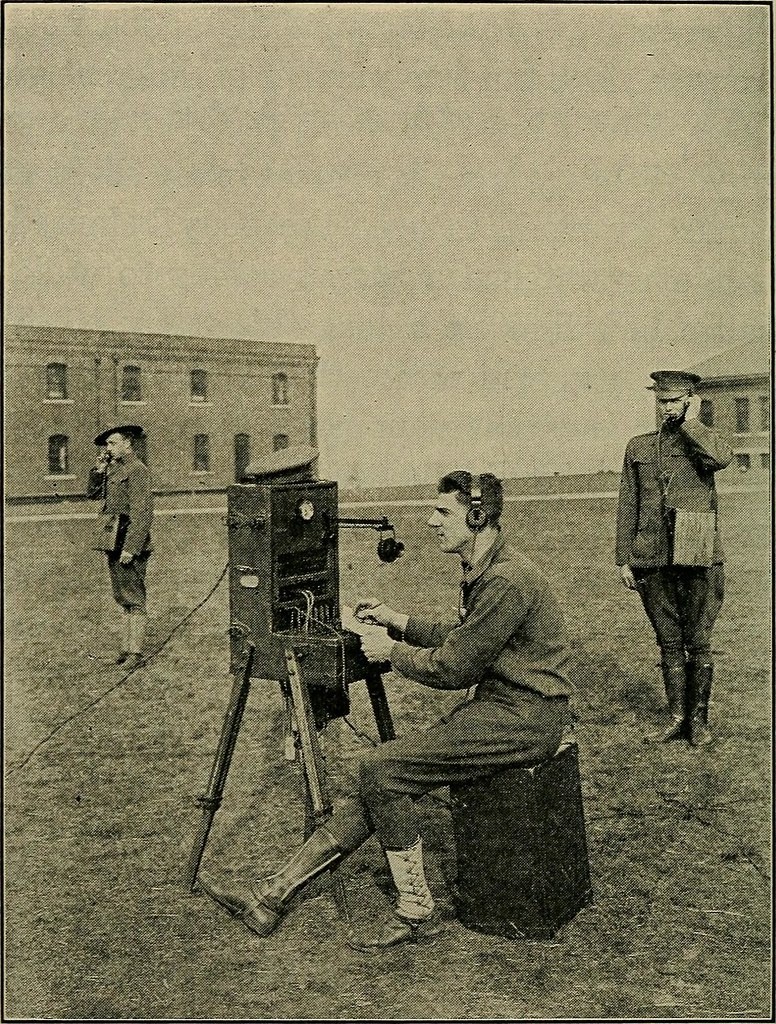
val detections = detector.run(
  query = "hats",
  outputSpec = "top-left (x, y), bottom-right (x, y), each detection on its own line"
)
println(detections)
top-left (244, 445), bottom-right (322, 485)
top-left (650, 371), bottom-right (702, 400)
top-left (92, 417), bottom-right (147, 447)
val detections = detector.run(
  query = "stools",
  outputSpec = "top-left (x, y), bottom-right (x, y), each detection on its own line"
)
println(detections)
top-left (450, 740), bottom-right (592, 941)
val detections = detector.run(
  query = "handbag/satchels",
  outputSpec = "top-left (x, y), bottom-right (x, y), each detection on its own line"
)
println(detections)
top-left (672, 510), bottom-right (716, 567)
top-left (90, 512), bottom-right (120, 551)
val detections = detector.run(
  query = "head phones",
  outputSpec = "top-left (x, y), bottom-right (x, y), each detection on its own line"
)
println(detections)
top-left (465, 475), bottom-right (489, 532)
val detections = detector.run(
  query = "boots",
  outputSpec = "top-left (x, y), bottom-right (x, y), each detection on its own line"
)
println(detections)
top-left (121, 616), bottom-right (146, 670)
top-left (196, 827), bottom-right (342, 937)
top-left (102, 614), bottom-right (130, 665)
top-left (349, 834), bottom-right (446, 952)
top-left (643, 663), bottom-right (689, 742)
top-left (685, 663), bottom-right (717, 750)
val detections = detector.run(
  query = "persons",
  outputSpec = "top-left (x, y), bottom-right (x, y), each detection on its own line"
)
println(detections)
top-left (615, 371), bottom-right (735, 748)
top-left (84, 424), bottom-right (154, 672)
top-left (198, 470), bottom-right (580, 954)
